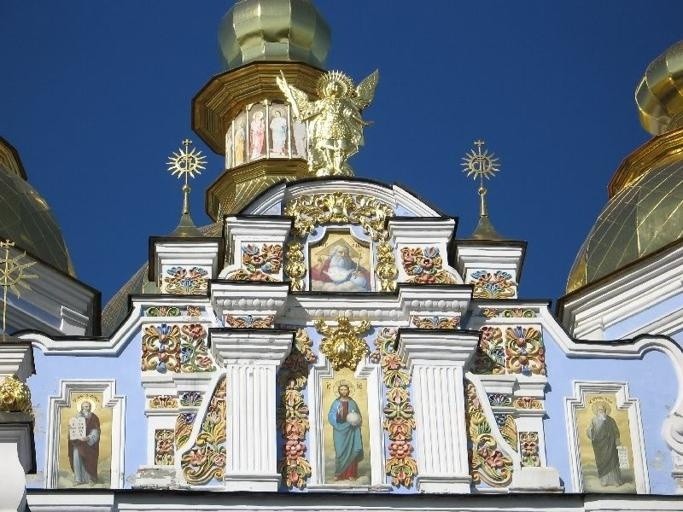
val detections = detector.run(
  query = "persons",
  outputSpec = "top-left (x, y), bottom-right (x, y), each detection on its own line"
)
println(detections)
top-left (270, 111), bottom-right (286, 153)
top-left (587, 405), bottom-right (622, 487)
top-left (251, 112), bottom-right (265, 160)
top-left (320, 245), bottom-right (369, 288)
top-left (68, 401), bottom-right (100, 486)
top-left (328, 385), bottom-right (363, 482)
top-left (235, 121), bottom-right (245, 166)
top-left (300, 81), bottom-right (369, 175)
